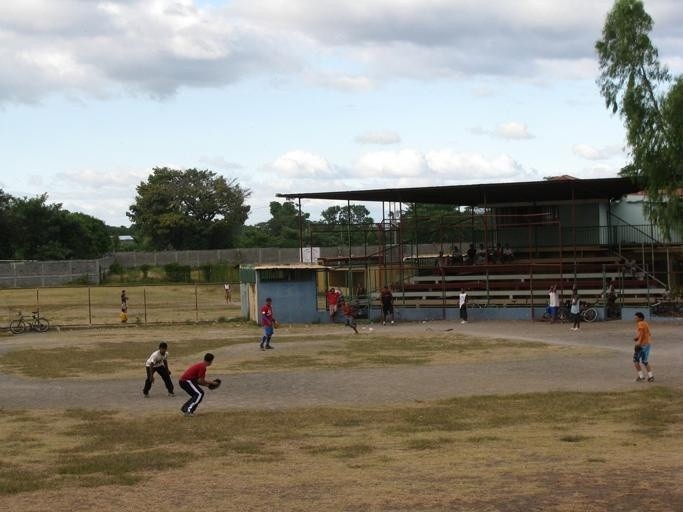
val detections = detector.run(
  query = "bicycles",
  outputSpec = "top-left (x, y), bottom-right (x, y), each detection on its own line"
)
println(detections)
top-left (9, 311), bottom-right (50, 334)
top-left (542, 300), bottom-right (598, 323)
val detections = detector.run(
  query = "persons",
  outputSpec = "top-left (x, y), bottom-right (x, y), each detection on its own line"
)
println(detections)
top-left (142, 342), bottom-right (174, 396)
top-left (606, 280), bottom-right (617, 318)
top-left (569, 287), bottom-right (580, 331)
top-left (434, 243), bottom-right (513, 274)
top-left (620, 255), bottom-right (638, 278)
top-left (258, 298), bottom-right (279, 350)
top-left (120, 306), bottom-right (128, 322)
top-left (119, 289), bottom-right (129, 306)
top-left (456, 286), bottom-right (468, 323)
top-left (223, 281), bottom-right (232, 304)
top-left (355, 283), bottom-right (365, 296)
top-left (325, 288), bottom-right (343, 324)
top-left (338, 297), bottom-right (358, 334)
top-left (632, 312), bottom-right (655, 381)
top-left (380, 285), bottom-right (394, 325)
top-left (545, 284), bottom-right (559, 324)
top-left (178, 352), bottom-right (221, 416)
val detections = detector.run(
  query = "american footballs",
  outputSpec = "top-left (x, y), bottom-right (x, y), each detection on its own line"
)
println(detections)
top-left (208, 380), bottom-right (221, 389)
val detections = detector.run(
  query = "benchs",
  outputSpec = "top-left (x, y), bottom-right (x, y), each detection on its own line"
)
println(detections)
top-left (373, 248), bottom-right (668, 307)
